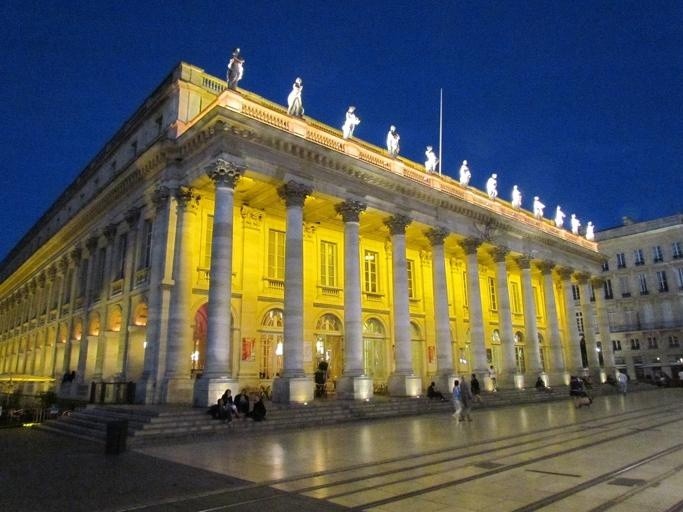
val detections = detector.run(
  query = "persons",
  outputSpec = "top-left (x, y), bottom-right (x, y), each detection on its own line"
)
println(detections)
top-left (570, 375), bottom-right (591, 407)
top-left (216, 388), bottom-right (266, 423)
top-left (424, 144), bottom-right (439, 173)
top-left (536, 377), bottom-right (552, 393)
top-left (460, 159), bottom-right (595, 243)
top-left (487, 365), bottom-right (498, 393)
top-left (386, 124), bottom-right (400, 154)
top-left (228, 46), bottom-right (244, 83)
top-left (619, 370), bottom-right (628, 394)
top-left (448, 373), bottom-right (483, 422)
top-left (427, 381), bottom-right (447, 403)
top-left (288, 76), bottom-right (304, 109)
top-left (342, 105), bottom-right (362, 138)
